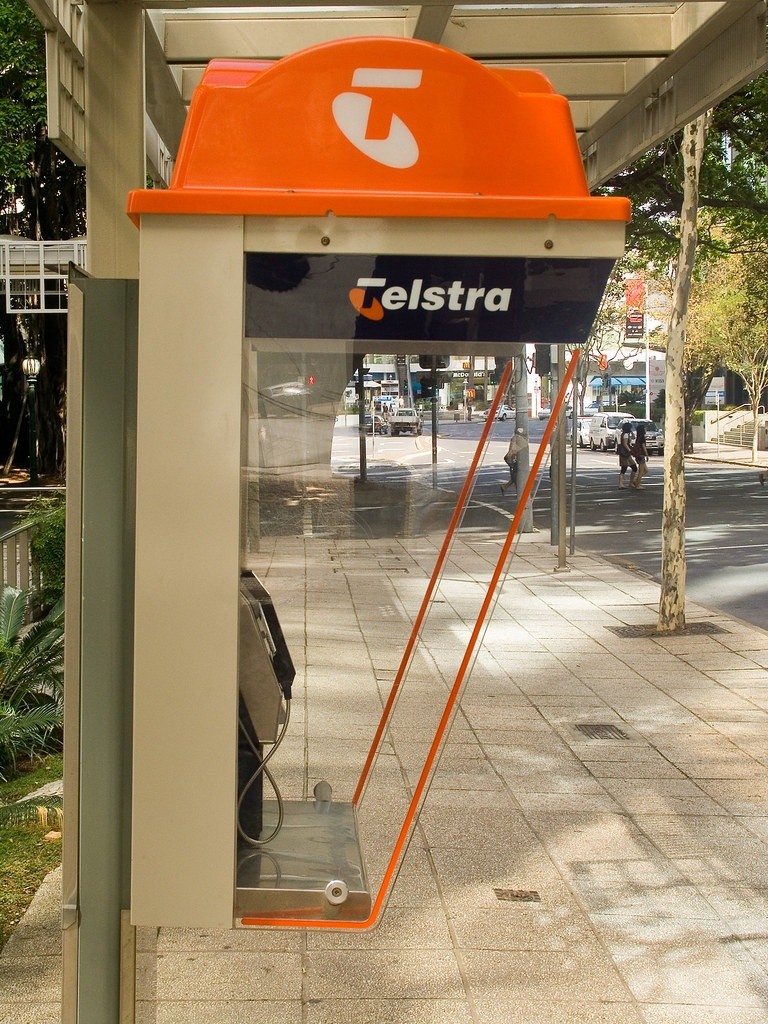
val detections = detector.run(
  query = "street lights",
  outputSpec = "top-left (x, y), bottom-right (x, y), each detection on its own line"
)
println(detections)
top-left (463, 379), bottom-right (468, 422)
top-left (23, 342), bottom-right (42, 489)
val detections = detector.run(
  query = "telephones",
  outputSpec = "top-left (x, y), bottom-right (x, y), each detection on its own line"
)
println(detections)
top-left (236, 569), bottom-right (296, 743)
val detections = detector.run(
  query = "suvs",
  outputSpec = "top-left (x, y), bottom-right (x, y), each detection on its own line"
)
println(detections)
top-left (614, 418), bottom-right (664, 457)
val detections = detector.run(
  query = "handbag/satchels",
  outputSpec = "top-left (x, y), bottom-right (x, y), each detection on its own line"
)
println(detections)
top-left (630, 442), bottom-right (646, 457)
top-left (504, 450), bottom-right (517, 468)
top-left (616, 444), bottom-right (632, 456)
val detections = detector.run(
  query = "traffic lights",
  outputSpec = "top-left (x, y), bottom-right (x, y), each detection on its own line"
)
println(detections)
top-left (403, 380), bottom-right (409, 389)
top-left (489, 372), bottom-right (498, 385)
top-left (361, 367), bottom-right (370, 376)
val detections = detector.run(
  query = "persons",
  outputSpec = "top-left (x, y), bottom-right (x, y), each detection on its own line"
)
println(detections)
top-left (398, 396), bottom-right (404, 408)
top-left (759, 474), bottom-right (768, 486)
top-left (619, 423), bottom-right (638, 490)
top-left (389, 403), bottom-right (393, 416)
top-left (633, 425), bottom-right (650, 489)
top-left (500, 437), bottom-right (517, 497)
top-left (383, 403), bottom-right (388, 421)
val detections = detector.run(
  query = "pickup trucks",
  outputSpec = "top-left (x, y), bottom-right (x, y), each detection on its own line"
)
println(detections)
top-left (388, 408), bottom-right (424, 437)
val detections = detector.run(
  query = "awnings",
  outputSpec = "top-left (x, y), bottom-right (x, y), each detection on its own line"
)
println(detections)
top-left (589, 375), bottom-right (647, 388)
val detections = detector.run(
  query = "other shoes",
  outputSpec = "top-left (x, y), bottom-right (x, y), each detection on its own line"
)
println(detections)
top-left (629, 484), bottom-right (635, 488)
top-left (758, 474), bottom-right (766, 485)
top-left (618, 486), bottom-right (626, 489)
top-left (500, 483), bottom-right (507, 495)
top-left (636, 486), bottom-right (645, 490)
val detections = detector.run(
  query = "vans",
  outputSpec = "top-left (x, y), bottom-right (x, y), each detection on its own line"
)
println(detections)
top-left (589, 412), bottom-right (635, 452)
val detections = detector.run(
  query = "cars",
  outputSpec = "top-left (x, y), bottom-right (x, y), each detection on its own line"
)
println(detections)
top-left (567, 417), bottom-right (593, 448)
top-left (537, 404), bottom-right (574, 420)
top-left (584, 403), bottom-right (598, 416)
top-left (366, 415), bottom-right (389, 436)
top-left (482, 404), bottom-right (516, 422)
